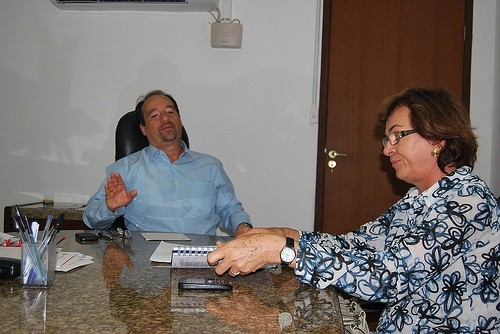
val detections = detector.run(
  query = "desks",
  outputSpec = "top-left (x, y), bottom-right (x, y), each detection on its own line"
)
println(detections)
top-left (0, 229), bottom-right (372, 333)
top-left (3, 201), bottom-right (87, 229)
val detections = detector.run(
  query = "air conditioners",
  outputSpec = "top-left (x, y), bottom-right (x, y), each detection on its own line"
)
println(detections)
top-left (49, 0), bottom-right (220, 12)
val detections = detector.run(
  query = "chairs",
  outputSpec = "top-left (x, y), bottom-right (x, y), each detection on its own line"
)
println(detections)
top-left (111, 109), bottom-right (190, 229)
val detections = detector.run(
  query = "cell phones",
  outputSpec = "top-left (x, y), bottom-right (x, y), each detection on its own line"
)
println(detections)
top-left (178, 277), bottom-right (233, 291)
top-left (75, 233), bottom-right (99, 241)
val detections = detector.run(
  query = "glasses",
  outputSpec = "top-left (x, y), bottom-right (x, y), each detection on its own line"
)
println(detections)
top-left (381, 127), bottom-right (417, 147)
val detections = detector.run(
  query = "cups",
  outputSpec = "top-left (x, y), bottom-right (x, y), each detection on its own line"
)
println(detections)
top-left (21, 244), bottom-right (56, 288)
top-left (23, 286), bottom-right (47, 332)
top-left (43, 194), bottom-right (54, 206)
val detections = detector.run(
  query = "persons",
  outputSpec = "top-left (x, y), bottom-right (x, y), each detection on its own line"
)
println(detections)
top-left (202, 285), bottom-right (369, 334)
top-left (102, 241), bottom-right (134, 290)
top-left (82, 90), bottom-right (253, 239)
top-left (207, 86), bottom-right (500, 334)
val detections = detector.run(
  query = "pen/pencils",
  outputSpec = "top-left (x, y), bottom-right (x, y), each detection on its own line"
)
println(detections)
top-left (12, 205), bottom-right (66, 285)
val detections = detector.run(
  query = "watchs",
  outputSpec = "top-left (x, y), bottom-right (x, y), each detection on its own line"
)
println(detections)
top-left (237, 222), bottom-right (252, 229)
top-left (275, 301), bottom-right (293, 334)
top-left (280, 237), bottom-right (297, 268)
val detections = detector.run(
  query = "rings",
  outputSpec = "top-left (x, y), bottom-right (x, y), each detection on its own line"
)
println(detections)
top-left (231, 268), bottom-right (240, 276)
